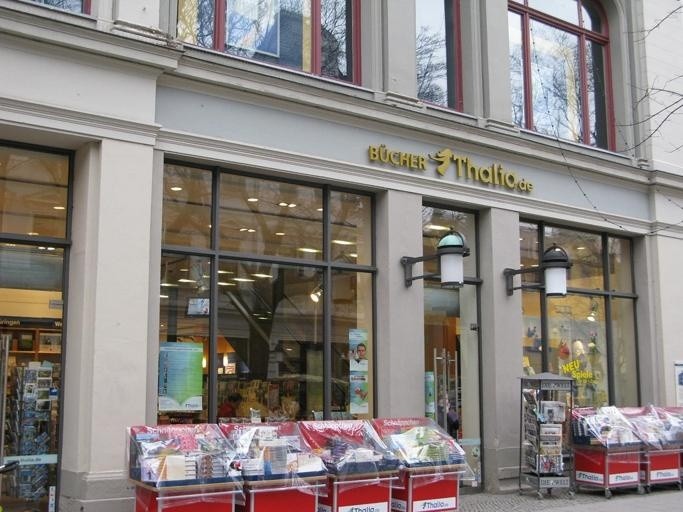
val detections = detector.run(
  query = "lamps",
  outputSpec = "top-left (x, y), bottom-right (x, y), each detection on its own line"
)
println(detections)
top-left (399, 227), bottom-right (470, 290)
top-left (503, 243), bottom-right (574, 298)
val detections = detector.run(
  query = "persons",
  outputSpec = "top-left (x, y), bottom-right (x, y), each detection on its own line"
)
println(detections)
top-left (218, 393), bottom-right (243, 418)
top-left (438, 393), bottom-right (459, 439)
top-left (350, 344), bottom-right (368, 363)
